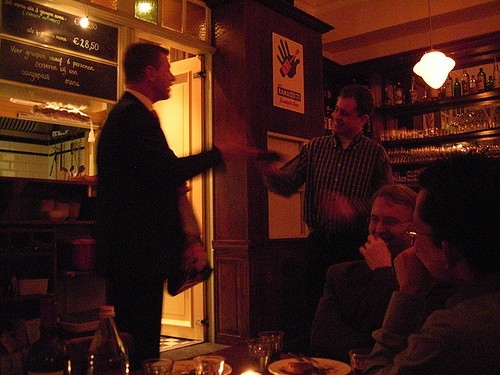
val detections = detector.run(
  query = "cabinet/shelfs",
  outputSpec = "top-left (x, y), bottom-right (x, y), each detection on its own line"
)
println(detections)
top-left (371, 87), bottom-right (500, 189)
top-left (0, 175), bottom-right (99, 323)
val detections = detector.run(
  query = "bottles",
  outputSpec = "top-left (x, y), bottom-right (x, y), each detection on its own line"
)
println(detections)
top-left (322, 86), bottom-right (338, 134)
top-left (87, 306), bottom-right (129, 375)
top-left (23, 324), bottom-right (72, 375)
top-left (382, 54), bottom-right (500, 107)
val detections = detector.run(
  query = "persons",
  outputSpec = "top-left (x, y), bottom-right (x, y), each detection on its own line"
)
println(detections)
top-left (368, 154), bottom-right (500, 375)
top-left (261, 84), bottom-right (392, 312)
top-left (310, 184), bottom-right (418, 355)
top-left (96, 42), bottom-right (258, 369)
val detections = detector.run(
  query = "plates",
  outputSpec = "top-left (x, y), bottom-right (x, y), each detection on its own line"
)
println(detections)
top-left (268, 356), bottom-right (351, 375)
top-left (173, 359), bottom-right (232, 375)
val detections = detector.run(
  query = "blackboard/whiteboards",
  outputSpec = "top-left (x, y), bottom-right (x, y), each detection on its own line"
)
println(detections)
top-left (0, 0), bottom-right (120, 105)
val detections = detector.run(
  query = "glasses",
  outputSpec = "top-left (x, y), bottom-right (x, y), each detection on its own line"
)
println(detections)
top-left (406, 225), bottom-right (464, 256)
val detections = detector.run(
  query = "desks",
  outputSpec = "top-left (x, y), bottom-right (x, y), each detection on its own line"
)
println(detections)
top-left (135, 342), bottom-right (276, 375)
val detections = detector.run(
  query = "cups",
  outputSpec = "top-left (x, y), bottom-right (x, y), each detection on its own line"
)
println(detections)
top-left (38, 197), bottom-right (80, 221)
top-left (349, 349), bottom-right (373, 375)
top-left (245, 338), bottom-right (273, 375)
top-left (193, 356), bottom-right (225, 375)
top-left (364, 104), bottom-right (500, 188)
top-left (258, 330), bottom-right (284, 361)
top-left (143, 358), bottom-right (174, 375)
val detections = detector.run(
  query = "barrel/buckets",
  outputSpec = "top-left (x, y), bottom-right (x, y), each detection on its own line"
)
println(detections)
top-left (69, 234), bottom-right (96, 270)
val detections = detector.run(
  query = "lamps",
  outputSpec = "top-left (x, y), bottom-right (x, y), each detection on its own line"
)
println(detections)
top-left (412, 0), bottom-right (456, 89)
